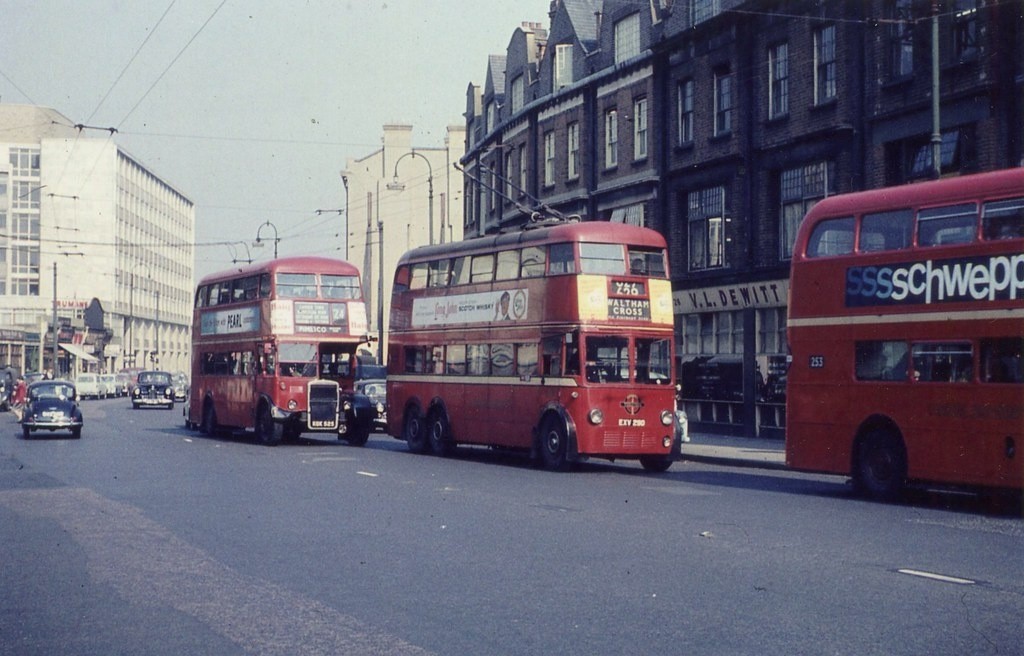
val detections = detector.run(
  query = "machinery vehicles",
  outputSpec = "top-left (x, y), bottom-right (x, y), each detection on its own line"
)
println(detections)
top-left (385, 158), bottom-right (682, 476)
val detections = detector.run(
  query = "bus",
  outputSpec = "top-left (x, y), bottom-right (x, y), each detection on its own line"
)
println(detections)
top-left (188, 220), bottom-right (379, 448)
top-left (784, 163), bottom-right (1023, 504)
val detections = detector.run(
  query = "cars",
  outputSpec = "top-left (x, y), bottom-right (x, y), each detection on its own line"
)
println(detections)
top-left (21, 379), bottom-right (84, 439)
top-left (170, 370), bottom-right (190, 404)
top-left (131, 371), bottom-right (178, 410)
top-left (0, 366), bottom-right (130, 413)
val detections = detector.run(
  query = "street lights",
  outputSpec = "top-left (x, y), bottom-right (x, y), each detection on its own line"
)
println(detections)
top-left (387, 148), bottom-right (434, 245)
top-left (127, 262), bottom-right (151, 376)
top-left (253, 220), bottom-right (279, 259)
